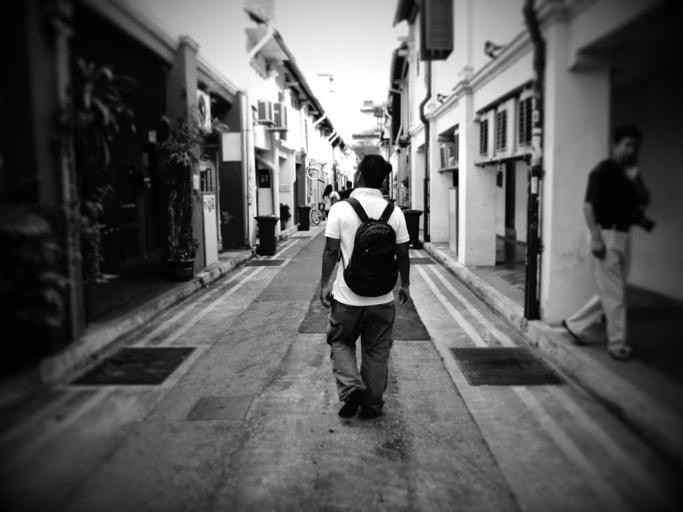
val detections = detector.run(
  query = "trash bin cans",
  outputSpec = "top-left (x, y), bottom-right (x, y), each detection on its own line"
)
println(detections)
top-left (404, 210), bottom-right (422, 241)
top-left (255, 216), bottom-right (278, 256)
top-left (297, 207), bottom-right (311, 230)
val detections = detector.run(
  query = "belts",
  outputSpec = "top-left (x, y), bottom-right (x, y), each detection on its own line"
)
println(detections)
top-left (601, 222), bottom-right (630, 231)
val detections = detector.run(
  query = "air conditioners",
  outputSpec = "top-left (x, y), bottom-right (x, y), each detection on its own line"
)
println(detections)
top-left (439, 142), bottom-right (456, 171)
top-left (268, 103), bottom-right (288, 132)
top-left (256, 96), bottom-right (275, 123)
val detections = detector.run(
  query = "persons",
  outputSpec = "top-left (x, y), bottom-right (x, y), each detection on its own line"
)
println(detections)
top-left (315, 155), bottom-right (411, 419)
top-left (319, 176), bottom-right (411, 236)
top-left (560, 128), bottom-right (655, 361)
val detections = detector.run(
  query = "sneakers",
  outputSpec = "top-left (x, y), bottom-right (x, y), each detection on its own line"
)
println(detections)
top-left (338, 389), bottom-right (366, 418)
top-left (359, 406), bottom-right (383, 419)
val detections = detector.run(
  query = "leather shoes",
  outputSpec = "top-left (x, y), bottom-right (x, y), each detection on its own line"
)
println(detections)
top-left (609, 344), bottom-right (631, 359)
top-left (562, 320), bottom-right (598, 345)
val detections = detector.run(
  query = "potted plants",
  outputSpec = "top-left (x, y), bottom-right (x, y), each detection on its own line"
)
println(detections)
top-left (155, 118), bottom-right (206, 283)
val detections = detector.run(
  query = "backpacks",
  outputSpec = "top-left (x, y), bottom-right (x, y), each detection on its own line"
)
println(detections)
top-left (337, 197), bottom-right (401, 297)
